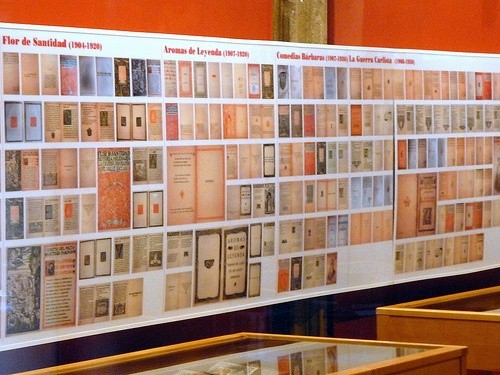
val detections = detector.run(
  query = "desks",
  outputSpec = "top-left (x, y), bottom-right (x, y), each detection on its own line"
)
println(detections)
top-left (9, 331), bottom-right (469, 375)
top-left (376, 286), bottom-right (500, 375)
